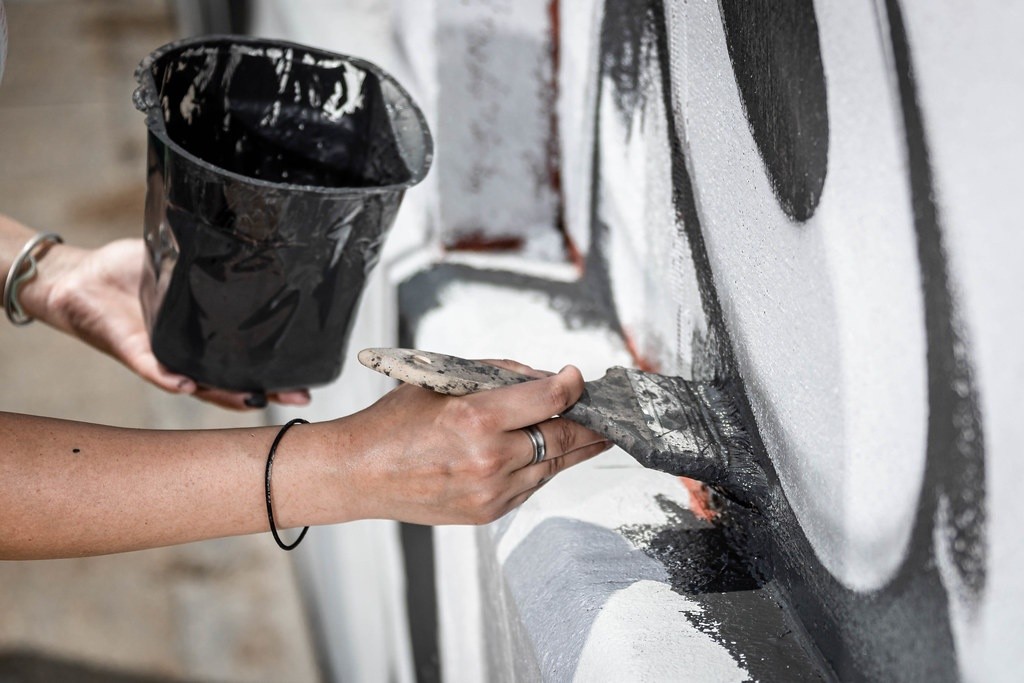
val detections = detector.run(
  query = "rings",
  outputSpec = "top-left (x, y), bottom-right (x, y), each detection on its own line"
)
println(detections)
top-left (522, 427), bottom-right (546, 465)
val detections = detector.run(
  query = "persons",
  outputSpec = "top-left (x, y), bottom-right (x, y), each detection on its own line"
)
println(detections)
top-left (0, 211), bottom-right (614, 560)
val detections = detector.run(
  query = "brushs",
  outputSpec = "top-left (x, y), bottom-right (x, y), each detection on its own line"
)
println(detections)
top-left (357, 348), bottom-right (774, 515)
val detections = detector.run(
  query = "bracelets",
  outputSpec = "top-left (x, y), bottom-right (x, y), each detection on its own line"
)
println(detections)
top-left (265, 418), bottom-right (309, 551)
top-left (2, 232), bottom-right (63, 325)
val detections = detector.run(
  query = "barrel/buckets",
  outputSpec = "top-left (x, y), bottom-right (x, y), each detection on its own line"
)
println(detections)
top-left (132, 35), bottom-right (434, 394)
